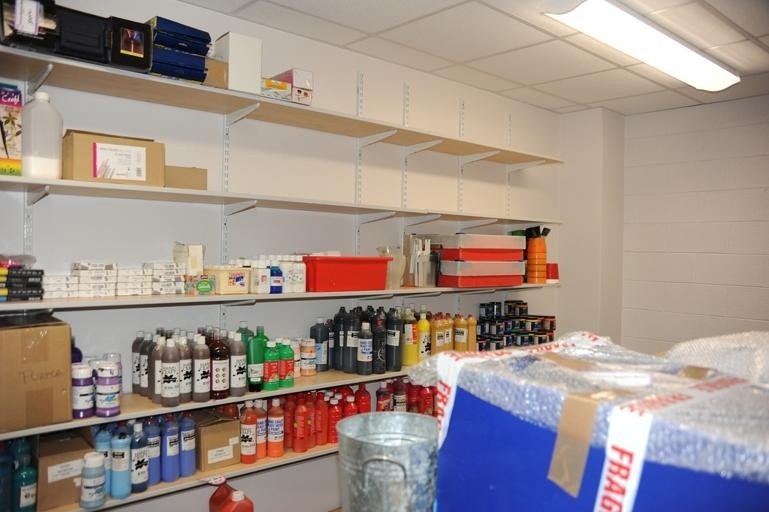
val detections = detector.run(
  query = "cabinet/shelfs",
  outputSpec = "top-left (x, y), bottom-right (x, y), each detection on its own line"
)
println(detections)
top-left (0, 46), bottom-right (562, 511)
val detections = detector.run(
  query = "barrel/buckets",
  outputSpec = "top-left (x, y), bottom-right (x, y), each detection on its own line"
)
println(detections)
top-left (209, 482), bottom-right (254, 511)
top-left (335, 411), bottom-right (441, 511)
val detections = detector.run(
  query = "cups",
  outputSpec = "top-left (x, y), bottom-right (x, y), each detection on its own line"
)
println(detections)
top-left (374, 246), bottom-right (404, 289)
top-left (528, 237), bottom-right (558, 282)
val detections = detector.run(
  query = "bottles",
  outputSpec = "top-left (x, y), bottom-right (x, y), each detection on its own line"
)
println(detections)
top-left (11, 306), bottom-right (476, 510)
top-left (234, 254), bottom-right (306, 294)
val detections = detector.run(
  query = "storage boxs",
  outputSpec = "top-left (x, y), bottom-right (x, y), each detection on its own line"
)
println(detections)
top-left (0, 316), bottom-right (103, 511)
top-left (60, 124), bottom-right (165, 186)
top-left (193, 412), bottom-right (241, 472)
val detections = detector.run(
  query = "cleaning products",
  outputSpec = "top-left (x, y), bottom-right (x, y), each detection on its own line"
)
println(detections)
top-left (80, 421), bottom-right (132, 508)
top-left (132, 306), bottom-right (478, 498)
